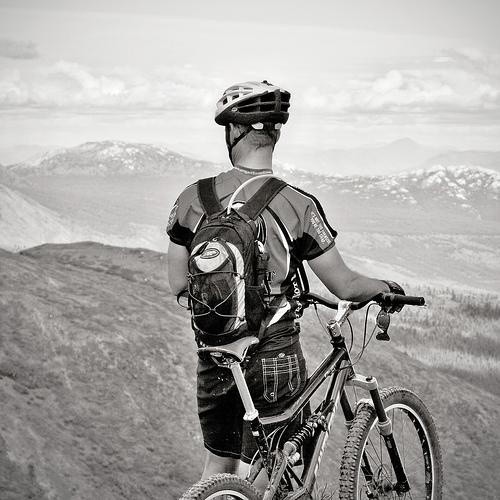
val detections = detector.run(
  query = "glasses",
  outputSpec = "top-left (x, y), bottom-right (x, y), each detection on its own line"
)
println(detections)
top-left (375, 306), bottom-right (392, 343)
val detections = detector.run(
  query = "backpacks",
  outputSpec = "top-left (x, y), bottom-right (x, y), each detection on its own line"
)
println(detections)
top-left (187, 176), bottom-right (289, 366)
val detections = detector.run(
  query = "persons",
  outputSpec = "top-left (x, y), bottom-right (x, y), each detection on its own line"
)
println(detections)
top-left (166, 78), bottom-right (405, 500)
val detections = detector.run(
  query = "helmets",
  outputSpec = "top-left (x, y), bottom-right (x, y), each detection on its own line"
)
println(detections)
top-left (213, 77), bottom-right (296, 126)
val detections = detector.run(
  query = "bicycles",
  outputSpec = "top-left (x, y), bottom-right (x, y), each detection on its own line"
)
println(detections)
top-left (180, 292), bottom-right (444, 500)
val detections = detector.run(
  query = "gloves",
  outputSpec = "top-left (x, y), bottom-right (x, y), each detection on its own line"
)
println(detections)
top-left (372, 280), bottom-right (408, 314)
